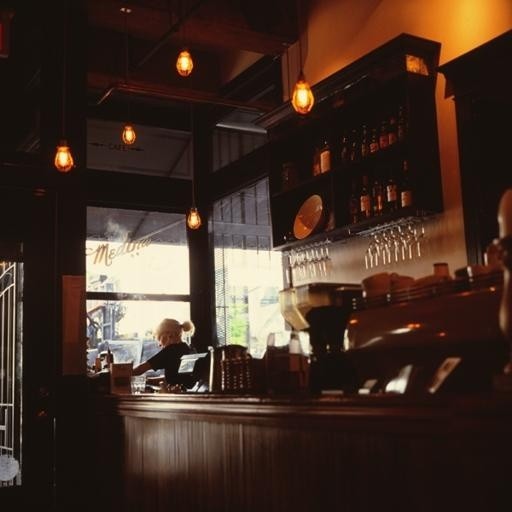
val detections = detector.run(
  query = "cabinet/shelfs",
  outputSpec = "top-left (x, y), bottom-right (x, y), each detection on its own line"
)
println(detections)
top-left (436, 27), bottom-right (512, 270)
top-left (249, 32), bottom-right (444, 255)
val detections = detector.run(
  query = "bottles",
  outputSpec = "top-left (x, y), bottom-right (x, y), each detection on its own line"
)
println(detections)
top-left (310, 101), bottom-right (413, 223)
top-left (91, 346), bottom-right (115, 375)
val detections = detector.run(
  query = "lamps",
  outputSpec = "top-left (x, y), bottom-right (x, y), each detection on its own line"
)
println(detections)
top-left (176, 0), bottom-right (194, 77)
top-left (53, 0), bottom-right (76, 174)
top-left (120, 0), bottom-right (137, 147)
top-left (292, 0), bottom-right (314, 115)
top-left (187, 101), bottom-right (202, 231)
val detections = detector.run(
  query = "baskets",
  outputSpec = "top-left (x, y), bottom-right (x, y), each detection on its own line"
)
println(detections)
top-left (219, 354), bottom-right (264, 395)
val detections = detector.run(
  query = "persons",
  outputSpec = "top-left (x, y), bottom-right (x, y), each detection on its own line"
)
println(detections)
top-left (132, 317), bottom-right (196, 389)
top-left (261, 332), bottom-right (276, 359)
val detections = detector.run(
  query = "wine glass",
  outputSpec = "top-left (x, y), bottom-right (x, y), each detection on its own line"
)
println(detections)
top-left (283, 241), bottom-right (335, 282)
top-left (362, 217), bottom-right (435, 270)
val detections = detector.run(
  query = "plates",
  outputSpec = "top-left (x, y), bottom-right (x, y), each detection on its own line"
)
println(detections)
top-left (292, 194), bottom-right (326, 241)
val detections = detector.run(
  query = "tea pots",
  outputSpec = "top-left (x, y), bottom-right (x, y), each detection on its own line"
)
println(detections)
top-left (218, 344), bottom-right (254, 395)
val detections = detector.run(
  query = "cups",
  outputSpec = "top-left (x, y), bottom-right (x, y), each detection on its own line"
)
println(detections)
top-left (129, 376), bottom-right (146, 396)
top-left (360, 260), bottom-right (503, 302)
